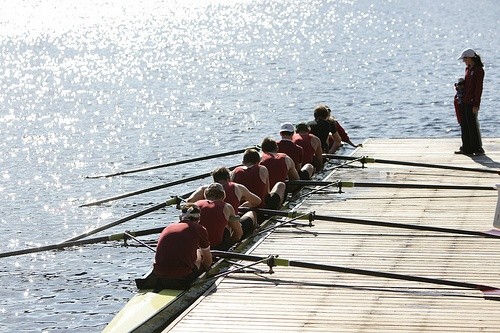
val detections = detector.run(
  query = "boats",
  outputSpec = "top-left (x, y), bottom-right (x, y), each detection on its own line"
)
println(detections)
top-left (99, 139), bottom-right (344, 333)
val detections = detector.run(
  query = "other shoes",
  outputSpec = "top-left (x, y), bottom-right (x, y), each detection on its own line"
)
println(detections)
top-left (474, 147), bottom-right (484, 155)
top-left (454, 146), bottom-right (469, 154)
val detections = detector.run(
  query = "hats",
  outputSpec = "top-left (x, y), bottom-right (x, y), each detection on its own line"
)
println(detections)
top-left (296, 121), bottom-right (308, 129)
top-left (280, 122), bottom-right (294, 133)
top-left (457, 48), bottom-right (475, 60)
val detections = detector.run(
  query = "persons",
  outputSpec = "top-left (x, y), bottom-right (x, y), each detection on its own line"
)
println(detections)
top-left (154, 202), bottom-right (213, 290)
top-left (272, 121), bottom-right (314, 194)
top-left (324, 105), bottom-right (363, 155)
top-left (257, 137), bottom-right (300, 201)
top-left (454, 48), bottom-right (486, 156)
top-left (305, 105), bottom-right (341, 161)
top-left (292, 122), bottom-right (323, 175)
top-left (230, 148), bottom-right (286, 221)
top-left (181, 166), bottom-right (262, 247)
top-left (187, 182), bottom-right (243, 255)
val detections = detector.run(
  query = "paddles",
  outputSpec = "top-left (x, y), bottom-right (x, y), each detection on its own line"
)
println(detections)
top-left (208, 246), bottom-right (496, 302)
top-left (61, 190), bottom-right (198, 243)
top-left (86, 141), bottom-right (266, 179)
top-left (0, 226), bottom-right (165, 261)
top-left (237, 203), bottom-right (496, 241)
top-left (320, 151), bottom-right (496, 176)
top-left (79, 165), bottom-right (244, 210)
top-left (285, 175), bottom-right (496, 192)
